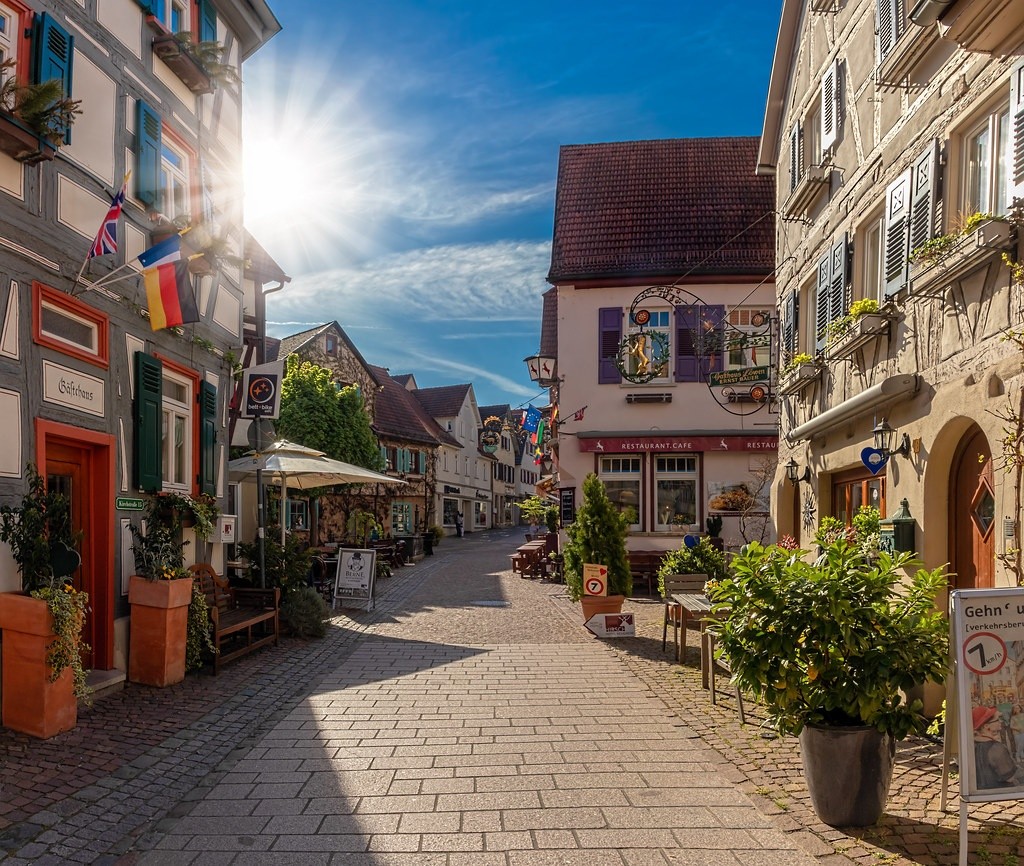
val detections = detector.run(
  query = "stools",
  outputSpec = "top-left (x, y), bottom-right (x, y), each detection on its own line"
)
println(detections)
top-left (512, 553), bottom-right (527, 573)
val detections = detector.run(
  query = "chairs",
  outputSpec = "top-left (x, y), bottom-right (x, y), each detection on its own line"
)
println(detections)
top-left (705, 630), bottom-right (745, 726)
top-left (307, 556), bottom-right (333, 608)
top-left (663, 574), bottom-right (709, 663)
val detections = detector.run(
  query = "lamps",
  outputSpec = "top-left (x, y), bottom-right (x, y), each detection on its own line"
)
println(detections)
top-left (784, 457), bottom-right (810, 487)
top-left (523, 350), bottom-right (565, 389)
top-left (869, 417), bottom-right (910, 463)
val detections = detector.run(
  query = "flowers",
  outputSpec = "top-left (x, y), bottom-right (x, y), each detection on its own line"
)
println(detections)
top-left (125, 489), bottom-right (220, 674)
top-left (0, 458), bottom-right (96, 707)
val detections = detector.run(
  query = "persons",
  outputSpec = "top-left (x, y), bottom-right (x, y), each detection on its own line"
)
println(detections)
top-left (454, 511), bottom-right (464, 536)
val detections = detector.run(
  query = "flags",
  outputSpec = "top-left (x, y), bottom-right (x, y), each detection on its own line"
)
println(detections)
top-left (240, 359), bottom-right (284, 419)
top-left (136, 231), bottom-right (182, 270)
top-left (573, 408), bottom-right (585, 422)
top-left (142, 256), bottom-right (200, 332)
top-left (519, 401), bottom-right (558, 465)
top-left (85, 182), bottom-right (127, 259)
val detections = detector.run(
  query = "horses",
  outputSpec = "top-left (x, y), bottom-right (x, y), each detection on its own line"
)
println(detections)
top-left (623, 335), bottom-right (651, 374)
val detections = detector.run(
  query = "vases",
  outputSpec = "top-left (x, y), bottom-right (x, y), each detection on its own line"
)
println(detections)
top-left (127, 576), bottom-right (193, 687)
top-left (0, 591), bottom-right (83, 740)
top-left (420, 532), bottom-right (433, 555)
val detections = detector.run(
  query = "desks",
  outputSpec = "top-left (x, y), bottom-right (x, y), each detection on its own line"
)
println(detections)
top-left (516, 540), bottom-right (546, 579)
top-left (670, 593), bottom-right (734, 689)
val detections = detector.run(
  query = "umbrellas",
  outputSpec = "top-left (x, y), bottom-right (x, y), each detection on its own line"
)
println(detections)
top-left (228, 439), bottom-right (408, 632)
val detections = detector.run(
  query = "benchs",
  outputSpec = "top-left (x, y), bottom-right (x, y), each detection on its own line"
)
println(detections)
top-left (323, 556), bottom-right (338, 579)
top-left (338, 540), bottom-right (407, 569)
top-left (186, 563), bottom-right (280, 677)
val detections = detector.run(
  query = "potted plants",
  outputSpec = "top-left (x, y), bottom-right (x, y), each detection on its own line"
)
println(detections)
top-left (0, 57), bottom-right (83, 167)
top-left (822, 298), bottom-right (882, 362)
top-left (905, 203), bottom-right (1011, 296)
top-left (562, 472), bottom-right (633, 623)
top-left (148, 214), bottom-right (252, 278)
top-left (702, 505), bottom-right (951, 826)
top-left (428, 526), bottom-right (445, 547)
top-left (779, 352), bottom-right (815, 394)
top-left (513, 494), bottom-right (548, 543)
top-left (151, 31), bottom-right (244, 96)
top-left (543, 502), bottom-right (560, 558)
